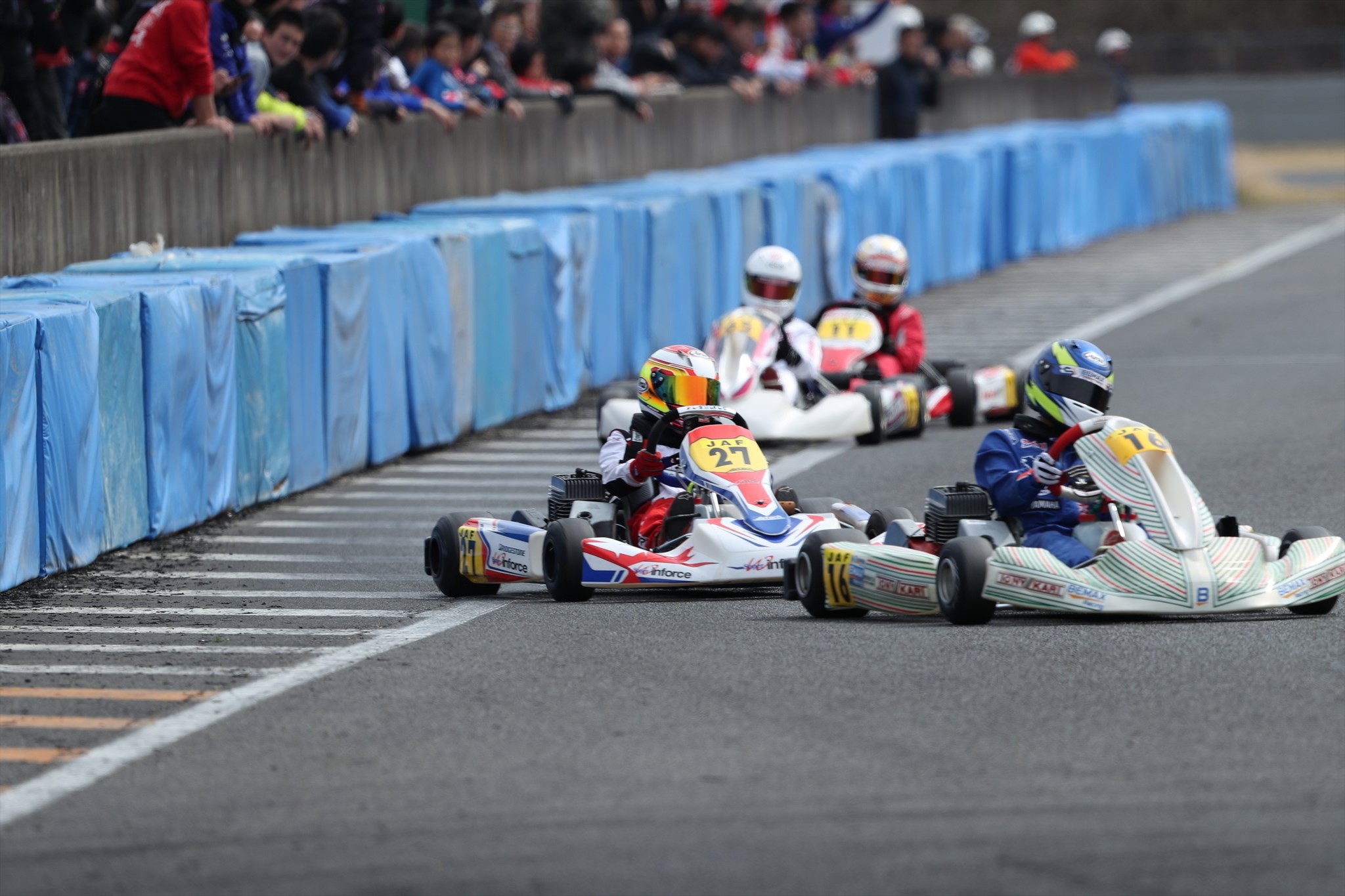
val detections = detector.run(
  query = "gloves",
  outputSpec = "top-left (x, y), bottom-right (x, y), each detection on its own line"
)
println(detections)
top-left (630, 449), bottom-right (664, 483)
top-left (1033, 452), bottom-right (1064, 485)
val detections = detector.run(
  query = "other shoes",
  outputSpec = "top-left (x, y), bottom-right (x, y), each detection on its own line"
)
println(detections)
top-left (657, 491), bottom-right (696, 553)
top-left (776, 485), bottom-right (798, 514)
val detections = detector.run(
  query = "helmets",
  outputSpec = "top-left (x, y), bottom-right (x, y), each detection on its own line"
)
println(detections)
top-left (1018, 12), bottom-right (1056, 40)
top-left (1025, 338), bottom-right (1115, 432)
top-left (637, 344), bottom-right (721, 434)
top-left (850, 232), bottom-right (910, 304)
top-left (741, 245), bottom-right (802, 321)
top-left (1096, 28), bottom-right (1131, 57)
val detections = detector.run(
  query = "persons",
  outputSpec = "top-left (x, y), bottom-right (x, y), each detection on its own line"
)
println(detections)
top-left (598, 344), bottom-right (799, 554)
top-left (973, 337), bottom-right (1152, 569)
top-left (702, 246), bottom-right (822, 411)
top-left (0, 0), bottom-right (1131, 152)
top-left (826, 233), bottom-right (924, 391)
